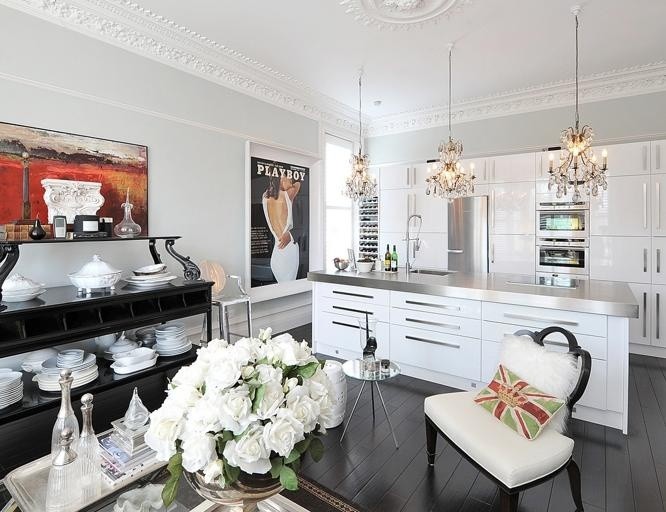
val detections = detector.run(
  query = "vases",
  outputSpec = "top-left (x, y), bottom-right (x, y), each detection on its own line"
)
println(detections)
top-left (182, 451), bottom-right (306, 512)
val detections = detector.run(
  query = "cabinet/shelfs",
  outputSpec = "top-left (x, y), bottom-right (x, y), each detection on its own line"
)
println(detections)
top-left (1, 278), bottom-right (215, 479)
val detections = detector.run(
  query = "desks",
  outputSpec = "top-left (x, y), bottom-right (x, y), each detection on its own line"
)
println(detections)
top-left (340, 356), bottom-right (401, 450)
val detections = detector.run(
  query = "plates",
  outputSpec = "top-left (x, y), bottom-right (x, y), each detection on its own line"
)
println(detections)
top-left (0, 256), bottom-right (195, 412)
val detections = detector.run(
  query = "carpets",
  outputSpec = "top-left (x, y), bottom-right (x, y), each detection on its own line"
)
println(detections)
top-left (279, 474), bottom-right (368, 512)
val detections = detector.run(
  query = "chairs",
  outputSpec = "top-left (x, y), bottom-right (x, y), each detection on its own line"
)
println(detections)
top-left (195, 260), bottom-right (253, 345)
top-left (424, 326), bottom-right (591, 512)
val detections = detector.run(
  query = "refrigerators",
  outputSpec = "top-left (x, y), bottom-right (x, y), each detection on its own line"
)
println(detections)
top-left (447, 196), bottom-right (489, 274)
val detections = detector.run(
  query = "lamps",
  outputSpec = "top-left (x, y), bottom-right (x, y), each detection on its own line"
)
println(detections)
top-left (341, 63), bottom-right (378, 203)
top-left (546, 6), bottom-right (609, 203)
top-left (425, 42), bottom-right (477, 202)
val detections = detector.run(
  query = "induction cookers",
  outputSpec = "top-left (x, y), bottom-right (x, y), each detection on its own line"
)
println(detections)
top-left (506, 274), bottom-right (583, 289)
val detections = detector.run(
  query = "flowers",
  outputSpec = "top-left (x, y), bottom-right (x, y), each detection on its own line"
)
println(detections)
top-left (143, 326), bottom-right (334, 509)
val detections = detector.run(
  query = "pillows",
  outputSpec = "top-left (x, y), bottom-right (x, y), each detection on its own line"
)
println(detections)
top-left (474, 365), bottom-right (565, 441)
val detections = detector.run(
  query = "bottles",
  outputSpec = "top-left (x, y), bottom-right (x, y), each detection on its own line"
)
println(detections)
top-left (42, 370), bottom-right (102, 511)
top-left (114, 206), bottom-right (141, 238)
top-left (391, 245), bottom-right (398, 272)
top-left (123, 386), bottom-right (148, 430)
top-left (385, 244), bottom-right (391, 271)
top-left (28, 219), bottom-right (47, 239)
top-left (361, 337), bottom-right (390, 373)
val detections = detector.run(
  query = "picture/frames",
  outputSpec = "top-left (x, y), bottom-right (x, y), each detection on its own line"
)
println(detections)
top-left (0, 122), bottom-right (148, 236)
top-left (244, 136), bottom-right (322, 304)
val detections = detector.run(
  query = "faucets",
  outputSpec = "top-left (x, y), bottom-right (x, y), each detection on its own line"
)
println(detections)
top-left (406, 214), bottom-right (423, 261)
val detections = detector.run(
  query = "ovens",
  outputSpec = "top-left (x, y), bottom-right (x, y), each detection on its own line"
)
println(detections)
top-left (538, 210), bottom-right (590, 275)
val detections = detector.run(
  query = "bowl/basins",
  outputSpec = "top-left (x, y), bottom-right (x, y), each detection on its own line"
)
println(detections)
top-left (356, 261), bottom-right (374, 273)
top-left (334, 262), bottom-right (349, 270)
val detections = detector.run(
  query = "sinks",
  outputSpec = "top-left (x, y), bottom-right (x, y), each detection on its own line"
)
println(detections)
top-left (410, 269), bottom-right (459, 276)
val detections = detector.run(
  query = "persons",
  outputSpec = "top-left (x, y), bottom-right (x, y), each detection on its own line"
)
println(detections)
top-left (262, 161), bottom-right (301, 283)
